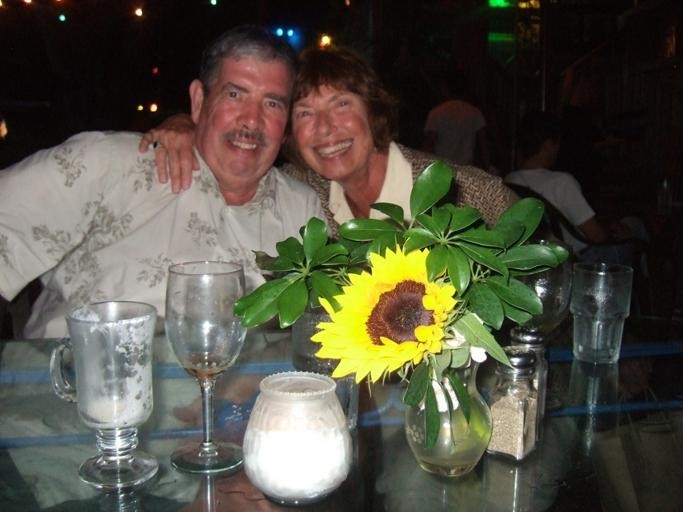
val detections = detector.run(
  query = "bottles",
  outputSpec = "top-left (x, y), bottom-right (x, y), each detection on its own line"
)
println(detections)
top-left (487, 346), bottom-right (541, 460)
top-left (243, 369), bottom-right (352, 505)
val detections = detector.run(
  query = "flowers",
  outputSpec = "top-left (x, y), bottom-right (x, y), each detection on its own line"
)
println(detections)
top-left (228, 160), bottom-right (568, 447)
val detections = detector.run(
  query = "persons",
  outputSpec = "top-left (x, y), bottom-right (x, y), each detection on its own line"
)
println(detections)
top-left (0, 22), bottom-right (331, 512)
top-left (424, 71), bottom-right (490, 171)
top-left (502, 112), bottom-right (653, 278)
top-left (137, 43), bottom-right (652, 512)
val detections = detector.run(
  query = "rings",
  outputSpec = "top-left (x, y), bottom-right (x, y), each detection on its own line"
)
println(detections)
top-left (153, 141), bottom-right (165, 149)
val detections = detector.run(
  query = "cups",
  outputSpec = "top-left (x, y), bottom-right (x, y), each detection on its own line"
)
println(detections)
top-left (290, 312), bottom-right (359, 431)
top-left (572, 257), bottom-right (632, 369)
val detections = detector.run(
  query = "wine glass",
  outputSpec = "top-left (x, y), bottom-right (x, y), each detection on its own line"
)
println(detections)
top-left (161, 258), bottom-right (246, 475)
top-left (510, 262), bottom-right (572, 411)
top-left (48, 298), bottom-right (160, 492)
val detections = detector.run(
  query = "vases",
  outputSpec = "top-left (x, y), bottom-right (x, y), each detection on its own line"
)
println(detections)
top-left (407, 341), bottom-right (493, 479)
